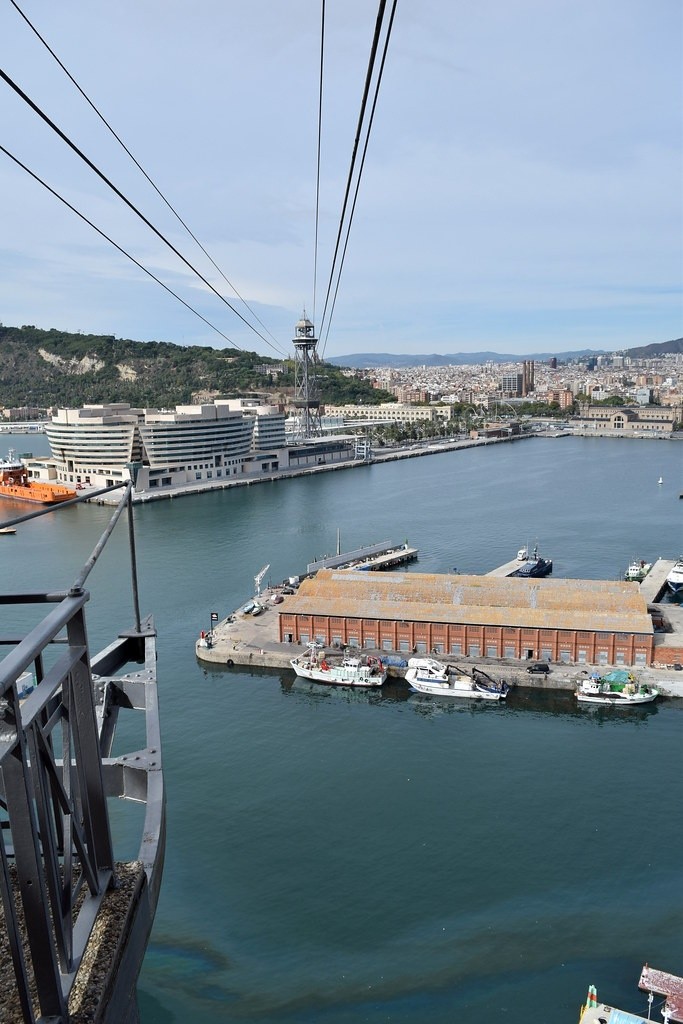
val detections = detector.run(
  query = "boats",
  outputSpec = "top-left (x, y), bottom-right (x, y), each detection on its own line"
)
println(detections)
top-left (243, 601), bottom-right (263, 616)
top-left (404, 657), bottom-right (511, 700)
top-left (288, 647), bottom-right (388, 686)
top-left (0, 449), bottom-right (78, 503)
top-left (667, 555), bottom-right (683, 594)
top-left (572, 672), bottom-right (659, 704)
top-left (624, 557), bottom-right (653, 582)
top-left (512, 537), bottom-right (553, 577)
top-left (0, 528), bottom-right (17, 533)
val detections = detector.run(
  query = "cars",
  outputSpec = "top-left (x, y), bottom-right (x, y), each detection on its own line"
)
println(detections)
top-left (281, 589), bottom-right (294, 595)
top-left (273, 597), bottom-right (284, 604)
top-left (526, 664), bottom-right (550, 674)
top-left (306, 642), bottom-right (324, 649)
